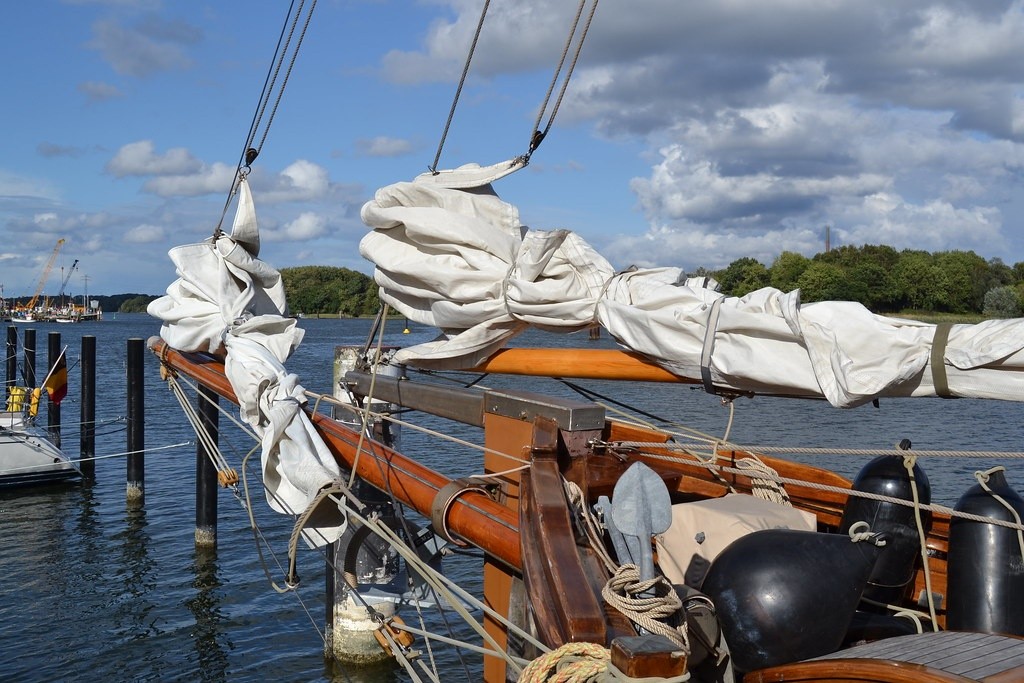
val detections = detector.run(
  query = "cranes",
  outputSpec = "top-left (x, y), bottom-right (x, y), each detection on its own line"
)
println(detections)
top-left (43, 259), bottom-right (80, 312)
top-left (12, 238), bottom-right (66, 314)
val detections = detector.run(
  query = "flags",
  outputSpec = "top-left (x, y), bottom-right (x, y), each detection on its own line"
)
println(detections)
top-left (42, 351), bottom-right (68, 406)
